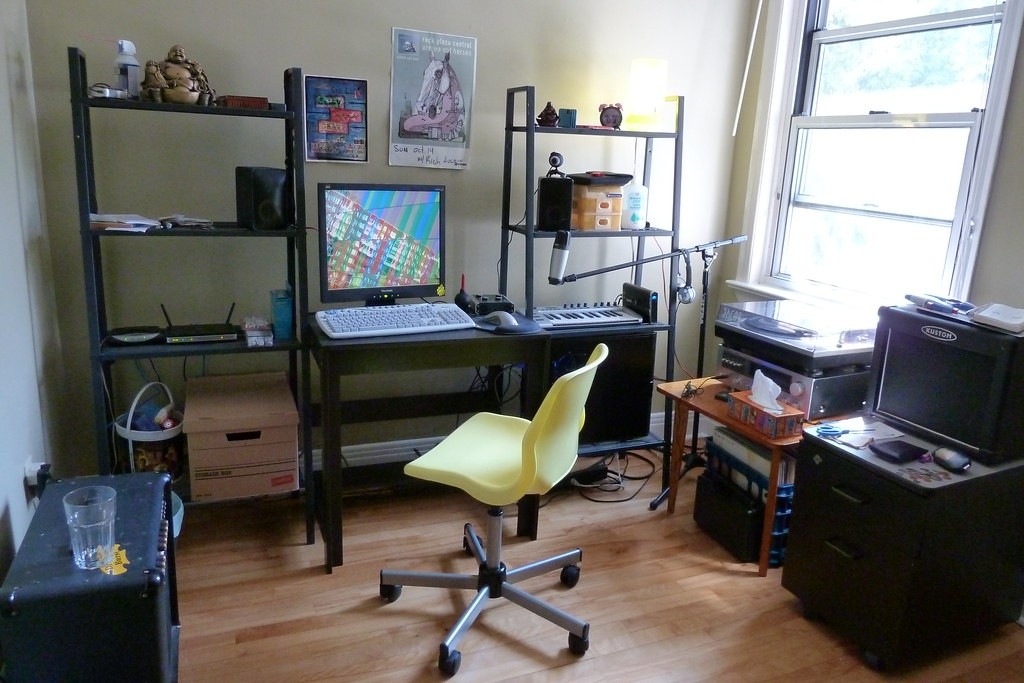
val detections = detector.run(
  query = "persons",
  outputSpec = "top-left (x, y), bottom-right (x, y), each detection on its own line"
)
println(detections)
top-left (143, 44), bottom-right (212, 93)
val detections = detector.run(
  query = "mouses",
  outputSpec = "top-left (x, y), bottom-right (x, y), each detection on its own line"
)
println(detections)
top-left (483, 311), bottom-right (519, 328)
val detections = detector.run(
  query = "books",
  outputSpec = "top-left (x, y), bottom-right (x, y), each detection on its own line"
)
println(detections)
top-left (970, 303), bottom-right (1024, 334)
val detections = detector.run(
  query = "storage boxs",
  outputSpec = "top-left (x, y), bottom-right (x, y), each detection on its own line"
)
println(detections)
top-left (571, 185), bottom-right (623, 231)
top-left (181, 373), bottom-right (299, 503)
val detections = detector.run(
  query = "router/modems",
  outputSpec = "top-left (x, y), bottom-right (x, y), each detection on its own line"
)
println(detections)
top-left (161, 303), bottom-right (238, 343)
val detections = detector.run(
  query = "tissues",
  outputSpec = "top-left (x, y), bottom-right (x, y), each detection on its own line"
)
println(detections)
top-left (726, 369), bottom-right (805, 440)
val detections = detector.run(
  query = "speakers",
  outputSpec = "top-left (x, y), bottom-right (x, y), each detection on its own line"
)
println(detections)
top-left (0, 470), bottom-right (179, 683)
top-left (537, 176), bottom-right (574, 233)
top-left (235, 166), bottom-right (296, 232)
top-left (865, 302), bottom-right (1024, 467)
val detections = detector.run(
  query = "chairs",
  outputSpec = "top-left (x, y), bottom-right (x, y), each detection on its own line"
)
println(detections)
top-left (379, 342), bottom-right (610, 677)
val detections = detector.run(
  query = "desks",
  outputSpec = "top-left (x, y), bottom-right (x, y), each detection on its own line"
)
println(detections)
top-left (656, 376), bottom-right (863, 578)
top-left (307, 302), bottom-right (551, 573)
top-left (0, 470), bottom-right (181, 683)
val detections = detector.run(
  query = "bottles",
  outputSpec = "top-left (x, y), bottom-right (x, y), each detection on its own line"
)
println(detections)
top-left (621, 166), bottom-right (649, 229)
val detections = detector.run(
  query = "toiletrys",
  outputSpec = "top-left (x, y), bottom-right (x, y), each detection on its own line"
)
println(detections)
top-left (621, 166), bottom-right (648, 230)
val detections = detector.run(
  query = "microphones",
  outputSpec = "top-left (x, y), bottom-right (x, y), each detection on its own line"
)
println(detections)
top-left (549, 230), bottom-right (571, 285)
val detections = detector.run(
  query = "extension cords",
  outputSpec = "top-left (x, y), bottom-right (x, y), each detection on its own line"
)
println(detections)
top-left (562, 465), bottom-right (608, 490)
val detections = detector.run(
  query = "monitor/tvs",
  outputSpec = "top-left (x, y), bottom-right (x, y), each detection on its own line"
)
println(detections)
top-left (318, 183), bottom-right (446, 308)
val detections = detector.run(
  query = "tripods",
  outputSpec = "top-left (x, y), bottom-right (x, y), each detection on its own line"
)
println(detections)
top-left (563, 234), bottom-right (750, 511)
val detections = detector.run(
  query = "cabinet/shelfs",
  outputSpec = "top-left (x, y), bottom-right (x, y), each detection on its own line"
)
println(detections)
top-left (499, 85), bottom-right (686, 491)
top-left (782, 421), bottom-right (1024, 670)
top-left (66, 47), bottom-right (315, 543)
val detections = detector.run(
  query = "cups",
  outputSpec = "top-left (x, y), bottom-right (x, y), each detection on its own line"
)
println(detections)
top-left (63, 486), bottom-right (116, 569)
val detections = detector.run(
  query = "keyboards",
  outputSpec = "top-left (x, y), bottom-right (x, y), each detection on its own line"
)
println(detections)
top-left (315, 304), bottom-right (476, 338)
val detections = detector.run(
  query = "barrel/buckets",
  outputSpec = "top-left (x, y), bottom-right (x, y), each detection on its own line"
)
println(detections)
top-left (114, 381), bottom-right (185, 486)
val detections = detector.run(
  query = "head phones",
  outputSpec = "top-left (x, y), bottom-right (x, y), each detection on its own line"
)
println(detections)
top-left (677, 248), bottom-right (696, 304)
